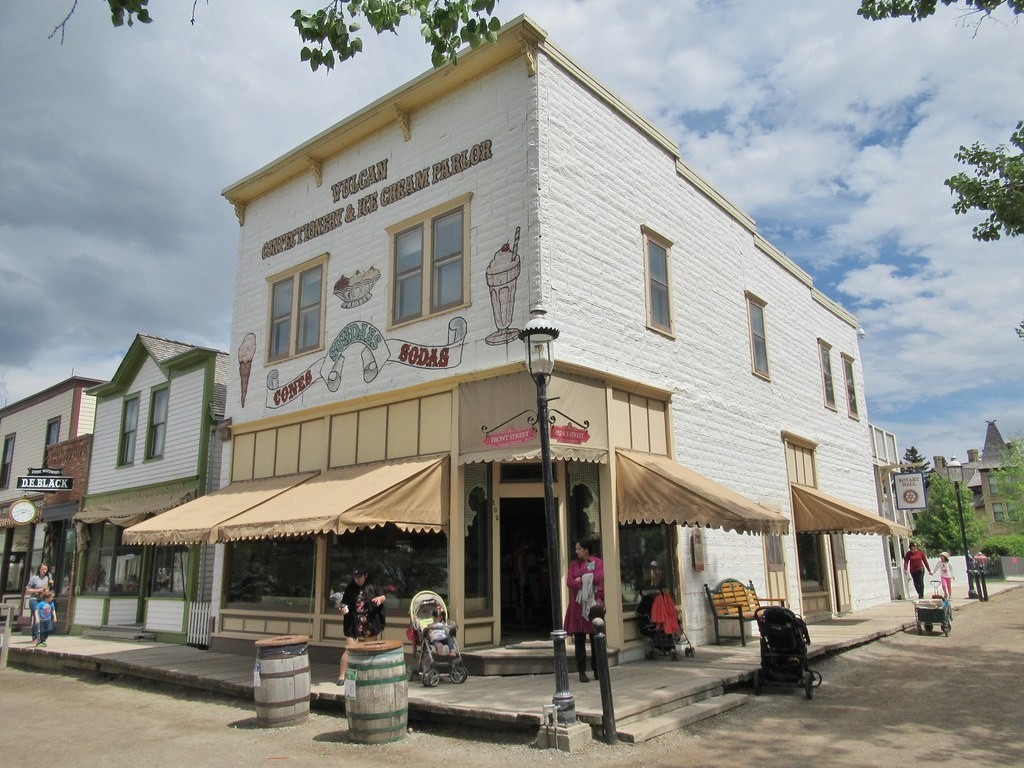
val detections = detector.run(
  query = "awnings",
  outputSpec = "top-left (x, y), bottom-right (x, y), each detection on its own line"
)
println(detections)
top-left (616, 450), bottom-right (790, 537)
top-left (121, 453), bottom-right (449, 546)
top-left (791, 484), bottom-right (908, 537)
top-left (72, 488), bottom-right (196, 553)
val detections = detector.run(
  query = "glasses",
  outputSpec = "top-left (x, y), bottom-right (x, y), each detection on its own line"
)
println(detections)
top-left (913, 545), bottom-right (917, 547)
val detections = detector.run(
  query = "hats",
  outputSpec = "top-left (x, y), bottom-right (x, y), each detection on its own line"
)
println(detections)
top-left (651, 561), bottom-right (657, 566)
top-left (352, 568), bottom-right (366, 577)
top-left (940, 552), bottom-right (949, 560)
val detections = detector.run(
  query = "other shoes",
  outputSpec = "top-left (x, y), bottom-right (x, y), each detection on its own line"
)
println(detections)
top-left (449, 649), bottom-right (456, 657)
top-left (948, 595), bottom-right (952, 599)
top-left (336, 678), bottom-right (345, 686)
top-left (579, 672), bottom-right (589, 682)
top-left (32, 639), bottom-right (39, 644)
top-left (35, 642), bottom-right (47, 647)
top-left (437, 643), bottom-right (444, 655)
top-left (594, 671), bottom-right (599, 680)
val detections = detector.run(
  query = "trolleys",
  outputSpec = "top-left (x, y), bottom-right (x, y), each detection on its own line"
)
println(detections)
top-left (912, 580), bottom-right (953, 637)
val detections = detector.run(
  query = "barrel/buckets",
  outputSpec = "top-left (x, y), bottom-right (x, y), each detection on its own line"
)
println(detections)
top-left (254, 635), bottom-right (312, 729)
top-left (345, 640), bottom-right (409, 744)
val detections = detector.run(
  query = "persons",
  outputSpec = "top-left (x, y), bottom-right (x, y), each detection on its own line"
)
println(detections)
top-left (977, 552), bottom-right (985, 567)
top-left (337, 564), bottom-right (388, 685)
top-left (26, 562), bottom-right (54, 643)
top-left (35, 591), bottom-right (57, 647)
top-left (932, 552), bottom-right (955, 601)
top-left (904, 541), bottom-right (932, 599)
top-left (564, 539), bottom-right (606, 682)
top-left (968, 550), bottom-right (975, 565)
top-left (427, 606), bottom-right (456, 657)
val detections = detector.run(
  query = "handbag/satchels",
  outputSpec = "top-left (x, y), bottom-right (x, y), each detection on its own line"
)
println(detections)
top-left (45, 574), bottom-right (53, 591)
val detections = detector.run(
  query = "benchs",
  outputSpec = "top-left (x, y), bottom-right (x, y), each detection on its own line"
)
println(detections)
top-left (705, 578), bottom-right (786, 645)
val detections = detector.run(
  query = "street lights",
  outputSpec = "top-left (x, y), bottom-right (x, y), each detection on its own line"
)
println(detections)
top-left (517, 304), bottom-right (593, 753)
top-left (945, 456), bottom-right (979, 599)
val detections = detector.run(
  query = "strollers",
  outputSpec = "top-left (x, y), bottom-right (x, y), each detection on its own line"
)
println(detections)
top-left (635, 585), bottom-right (695, 661)
top-left (754, 606), bottom-right (822, 700)
top-left (406, 590), bottom-right (467, 687)
